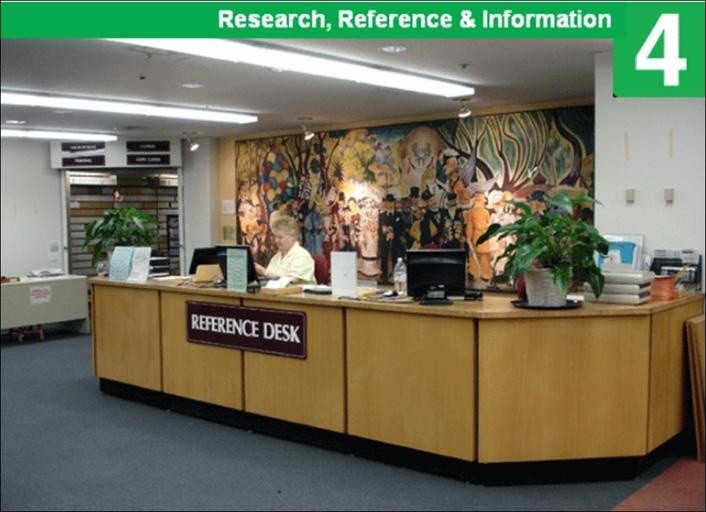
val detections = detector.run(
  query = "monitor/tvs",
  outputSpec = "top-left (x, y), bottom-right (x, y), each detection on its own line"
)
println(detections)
top-left (188, 247), bottom-right (215, 273)
top-left (404, 248), bottom-right (467, 305)
top-left (214, 245), bottom-right (261, 284)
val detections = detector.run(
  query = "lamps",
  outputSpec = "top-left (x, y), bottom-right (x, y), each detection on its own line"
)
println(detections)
top-left (182, 131), bottom-right (201, 152)
top-left (453, 97), bottom-right (474, 120)
top-left (98, 39), bottom-right (475, 100)
top-left (1, 89), bottom-right (258, 126)
top-left (296, 116), bottom-right (315, 141)
top-left (0, 127), bottom-right (117, 142)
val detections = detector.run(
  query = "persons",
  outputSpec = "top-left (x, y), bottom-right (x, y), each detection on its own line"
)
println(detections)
top-left (254, 215), bottom-right (317, 284)
top-left (320, 137), bottom-right (491, 286)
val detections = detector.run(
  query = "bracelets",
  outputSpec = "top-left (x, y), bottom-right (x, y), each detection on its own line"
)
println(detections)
top-left (263, 270), bottom-right (268, 277)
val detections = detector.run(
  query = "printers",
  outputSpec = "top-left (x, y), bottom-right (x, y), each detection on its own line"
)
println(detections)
top-left (650, 248), bottom-right (702, 283)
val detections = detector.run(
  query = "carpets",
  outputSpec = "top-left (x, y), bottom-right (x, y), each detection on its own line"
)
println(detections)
top-left (611, 456), bottom-right (706, 510)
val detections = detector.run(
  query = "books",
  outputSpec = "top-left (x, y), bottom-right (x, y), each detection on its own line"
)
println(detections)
top-left (584, 234), bottom-right (654, 306)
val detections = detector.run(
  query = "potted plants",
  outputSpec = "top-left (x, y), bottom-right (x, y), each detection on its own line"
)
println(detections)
top-left (477, 186), bottom-right (611, 312)
top-left (76, 207), bottom-right (162, 264)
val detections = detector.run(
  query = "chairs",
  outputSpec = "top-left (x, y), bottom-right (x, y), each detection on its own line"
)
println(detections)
top-left (4, 275), bottom-right (44, 341)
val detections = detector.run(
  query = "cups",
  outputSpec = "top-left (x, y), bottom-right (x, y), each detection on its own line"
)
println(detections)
top-left (648, 274), bottom-right (676, 302)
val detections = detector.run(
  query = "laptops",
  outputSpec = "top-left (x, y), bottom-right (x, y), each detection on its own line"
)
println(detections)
top-left (330, 250), bottom-right (376, 299)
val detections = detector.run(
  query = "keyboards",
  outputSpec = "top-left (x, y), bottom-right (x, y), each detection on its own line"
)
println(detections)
top-left (465, 290), bottom-right (483, 299)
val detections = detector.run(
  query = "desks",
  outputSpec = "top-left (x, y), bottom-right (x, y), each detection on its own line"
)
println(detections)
top-left (0, 271), bottom-right (90, 335)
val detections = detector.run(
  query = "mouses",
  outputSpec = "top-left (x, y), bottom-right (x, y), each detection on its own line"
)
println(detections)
top-left (383, 289), bottom-right (397, 297)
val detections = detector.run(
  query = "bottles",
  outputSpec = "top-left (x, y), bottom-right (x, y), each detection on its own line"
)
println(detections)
top-left (393, 257), bottom-right (408, 298)
